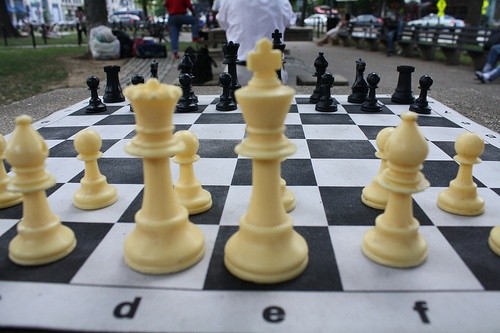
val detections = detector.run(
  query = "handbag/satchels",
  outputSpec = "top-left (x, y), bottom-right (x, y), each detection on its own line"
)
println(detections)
top-left (135, 43), bottom-right (167, 59)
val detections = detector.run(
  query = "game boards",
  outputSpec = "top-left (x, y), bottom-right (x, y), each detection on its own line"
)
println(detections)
top-left (1, 89), bottom-right (500, 333)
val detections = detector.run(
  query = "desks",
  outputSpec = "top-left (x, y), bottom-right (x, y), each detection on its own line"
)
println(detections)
top-left (208, 25), bottom-right (314, 41)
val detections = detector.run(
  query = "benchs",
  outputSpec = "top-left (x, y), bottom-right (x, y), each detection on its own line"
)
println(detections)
top-left (333, 20), bottom-right (497, 68)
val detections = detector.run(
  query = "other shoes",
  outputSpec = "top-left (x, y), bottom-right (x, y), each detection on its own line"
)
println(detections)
top-left (474, 70), bottom-right (492, 84)
top-left (193, 37), bottom-right (204, 43)
top-left (173, 52), bottom-right (180, 59)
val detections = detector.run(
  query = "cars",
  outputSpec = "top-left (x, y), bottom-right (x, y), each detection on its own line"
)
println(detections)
top-left (348, 15), bottom-right (383, 29)
top-left (406, 13), bottom-right (465, 30)
top-left (295, 5), bottom-right (340, 27)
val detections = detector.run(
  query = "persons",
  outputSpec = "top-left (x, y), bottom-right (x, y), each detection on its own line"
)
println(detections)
top-left (211, 0), bottom-right (298, 86)
top-left (473, 43), bottom-right (500, 87)
top-left (162, 0), bottom-right (205, 59)
top-left (371, 16), bottom-right (402, 57)
top-left (312, 9), bottom-right (349, 47)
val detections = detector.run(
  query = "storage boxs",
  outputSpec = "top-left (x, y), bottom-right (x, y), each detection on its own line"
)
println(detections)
top-left (136, 43), bottom-right (168, 57)
